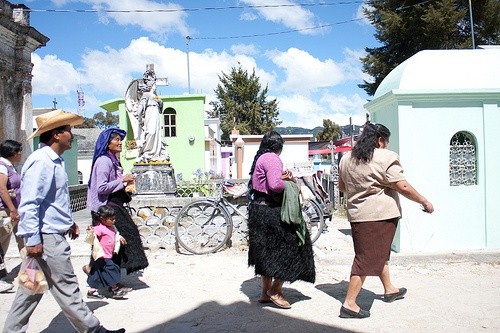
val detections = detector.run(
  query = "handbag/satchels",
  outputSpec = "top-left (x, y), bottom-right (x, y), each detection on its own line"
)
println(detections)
top-left (17, 255), bottom-right (50, 295)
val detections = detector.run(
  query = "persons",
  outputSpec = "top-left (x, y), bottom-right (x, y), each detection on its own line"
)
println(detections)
top-left (2, 109), bottom-right (125, 333)
top-left (248, 131), bottom-right (315, 309)
top-left (0, 140), bottom-right (27, 260)
top-left (82, 128), bottom-right (149, 293)
top-left (338, 123), bottom-right (434, 318)
top-left (85, 205), bottom-right (128, 299)
top-left (134, 81), bottom-right (168, 155)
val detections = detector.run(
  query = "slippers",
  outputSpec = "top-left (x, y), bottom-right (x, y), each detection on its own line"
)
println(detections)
top-left (265, 290), bottom-right (291, 308)
top-left (258, 295), bottom-right (273, 303)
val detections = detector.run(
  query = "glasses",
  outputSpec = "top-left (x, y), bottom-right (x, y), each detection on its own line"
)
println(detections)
top-left (17, 146), bottom-right (22, 151)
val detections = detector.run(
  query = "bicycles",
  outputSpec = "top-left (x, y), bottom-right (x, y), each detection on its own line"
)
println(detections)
top-left (174, 179), bottom-right (325, 255)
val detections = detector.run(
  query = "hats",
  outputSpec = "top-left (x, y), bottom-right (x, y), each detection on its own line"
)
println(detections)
top-left (26, 109), bottom-right (85, 140)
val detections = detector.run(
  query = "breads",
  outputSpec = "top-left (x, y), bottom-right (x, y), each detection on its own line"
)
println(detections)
top-left (16, 268), bottom-right (48, 292)
top-left (125, 180), bottom-right (135, 193)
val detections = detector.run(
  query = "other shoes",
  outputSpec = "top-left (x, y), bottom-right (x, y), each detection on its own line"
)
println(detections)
top-left (82, 265), bottom-right (91, 276)
top-left (340, 306), bottom-right (370, 318)
top-left (112, 288), bottom-right (126, 298)
top-left (76, 325), bottom-right (125, 333)
top-left (384, 288), bottom-right (407, 302)
top-left (87, 290), bottom-right (104, 299)
top-left (0, 280), bottom-right (16, 293)
top-left (119, 286), bottom-right (132, 291)
